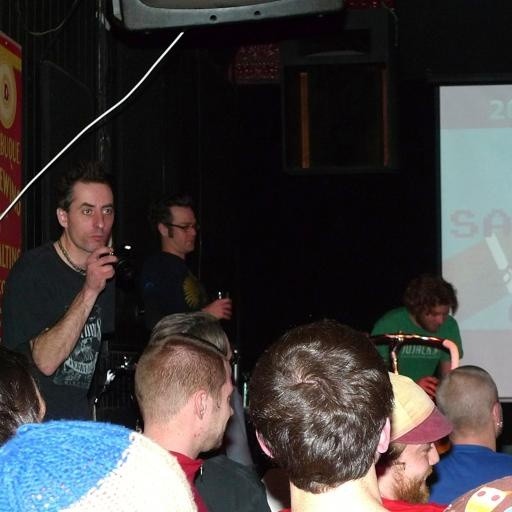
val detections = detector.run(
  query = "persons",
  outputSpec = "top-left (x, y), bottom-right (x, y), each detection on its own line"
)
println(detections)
top-left (0, 312), bottom-right (272, 512)
top-left (248, 320), bottom-right (510, 512)
top-left (135, 194), bottom-right (234, 331)
top-left (3, 162), bottom-right (119, 421)
top-left (369, 273), bottom-right (464, 399)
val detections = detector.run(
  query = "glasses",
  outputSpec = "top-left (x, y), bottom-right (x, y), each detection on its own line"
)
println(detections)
top-left (160, 221), bottom-right (200, 233)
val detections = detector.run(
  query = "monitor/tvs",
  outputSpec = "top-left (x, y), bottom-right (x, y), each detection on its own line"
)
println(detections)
top-left (123, 0), bottom-right (343, 30)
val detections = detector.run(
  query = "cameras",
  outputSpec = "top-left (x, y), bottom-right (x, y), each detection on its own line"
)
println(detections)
top-left (99, 251), bottom-right (149, 280)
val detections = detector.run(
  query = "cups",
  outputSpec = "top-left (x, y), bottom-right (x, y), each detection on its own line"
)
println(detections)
top-left (213, 288), bottom-right (232, 319)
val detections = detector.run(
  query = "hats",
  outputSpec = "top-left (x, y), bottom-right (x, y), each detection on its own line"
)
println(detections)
top-left (0, 418), bottom-right (208, 512)
top-left (387, 370), bottom-right (455, 446)
